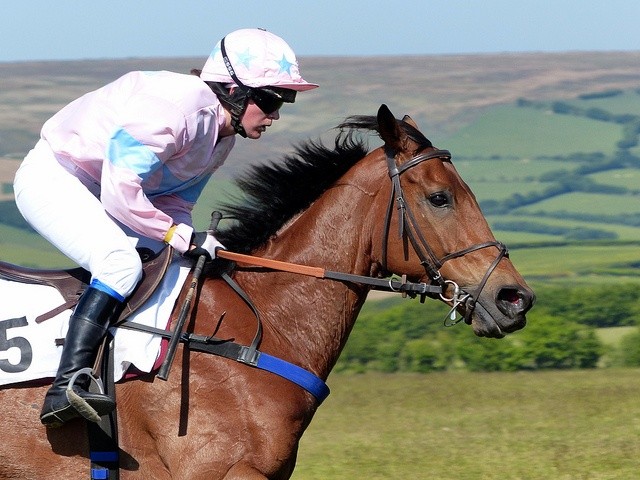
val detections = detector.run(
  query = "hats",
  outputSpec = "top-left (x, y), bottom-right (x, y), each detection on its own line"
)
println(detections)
top-left (200, 28), bottom-right (319, 91)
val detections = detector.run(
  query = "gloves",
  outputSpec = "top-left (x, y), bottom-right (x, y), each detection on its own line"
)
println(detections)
top-left (183, 231), bottom-right (227, 261)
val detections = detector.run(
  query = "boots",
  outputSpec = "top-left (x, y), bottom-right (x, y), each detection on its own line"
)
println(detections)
top-left (39, 287), bottom-right (124, 428)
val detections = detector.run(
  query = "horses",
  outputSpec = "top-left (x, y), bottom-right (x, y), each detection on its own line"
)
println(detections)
top-left (0, 102), bottom-right (536, 480)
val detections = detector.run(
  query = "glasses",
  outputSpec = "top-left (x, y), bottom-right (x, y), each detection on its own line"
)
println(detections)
top-left (221, 37), bottom-right (295, 115)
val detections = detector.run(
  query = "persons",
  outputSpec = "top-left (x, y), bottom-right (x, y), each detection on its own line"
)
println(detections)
top-left (12, 26), bottom-right (320, 430)
